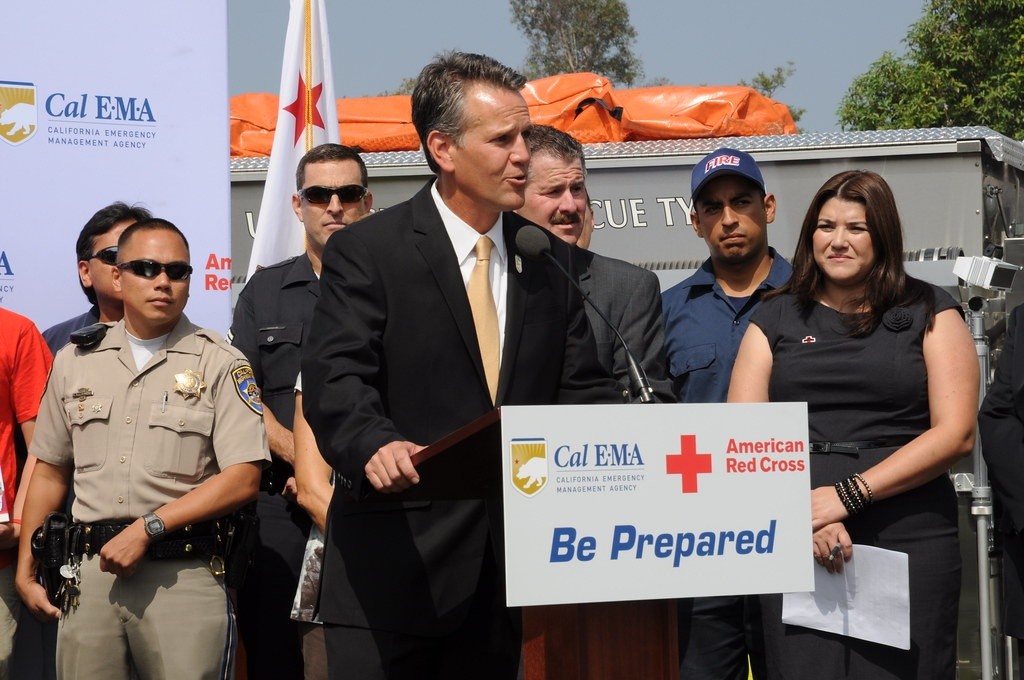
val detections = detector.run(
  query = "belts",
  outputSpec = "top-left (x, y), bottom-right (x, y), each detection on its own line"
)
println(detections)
top-left (76, 517), bottom-right (225, 559)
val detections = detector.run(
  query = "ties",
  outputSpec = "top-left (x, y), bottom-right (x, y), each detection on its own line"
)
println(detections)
top-left (465, 236), bottom-right (501, 407)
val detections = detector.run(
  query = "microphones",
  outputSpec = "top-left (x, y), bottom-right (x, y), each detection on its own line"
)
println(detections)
top-left (516, 226), bottom-right (663, 405)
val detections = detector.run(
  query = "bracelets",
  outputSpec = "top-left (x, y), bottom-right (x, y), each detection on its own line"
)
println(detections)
top-left (10, 519), bottom-right (22, 524)
top-left (834, 473), bottom-right (873, 515)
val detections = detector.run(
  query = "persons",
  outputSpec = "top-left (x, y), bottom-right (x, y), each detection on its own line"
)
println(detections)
top-left (0, 307), bottom-right (55, 679)
top-left (979, 302), bottom-right (1024, 680)
top-left (515, 127), bottom-right (677, 405)
top-left (657, 147), bottom-right (792, 680)
top-left (305, 50), bottom-right (631, 678)
top-left (229, 144), bottom-right (373, 679)
top-left (725, 169), bottom-right (981, 680)
top-left (41, 206), bottom-right (153, 357)
top-left (13, 219), bottom-right (273, 679)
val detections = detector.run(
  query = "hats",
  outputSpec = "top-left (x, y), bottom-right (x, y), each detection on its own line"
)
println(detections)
top-left (691, 148), bottom-right (765, 212)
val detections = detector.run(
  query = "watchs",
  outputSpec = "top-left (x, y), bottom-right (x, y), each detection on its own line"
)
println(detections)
top-left (142, 513), bottom-right (165, 541)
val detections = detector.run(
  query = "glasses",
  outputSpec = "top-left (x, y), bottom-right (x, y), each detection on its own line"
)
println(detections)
top-left (117, 259), bottom-right (193, 280)
top-left (299, 184), bottom-right (368, 204)
top-left (87, 246), bottom-right (118, 266)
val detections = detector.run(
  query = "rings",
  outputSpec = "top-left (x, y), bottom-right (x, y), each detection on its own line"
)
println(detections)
top-left (828, 555), bottom-right (834, 561)
top-left (831, 546), bottom-right (840, 555)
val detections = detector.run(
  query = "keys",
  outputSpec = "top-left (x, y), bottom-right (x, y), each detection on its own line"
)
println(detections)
top-left (55, 578), bottom-right (80, 612)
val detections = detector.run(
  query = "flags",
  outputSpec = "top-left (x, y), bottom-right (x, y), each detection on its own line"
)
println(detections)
top-left (246, 0), bottom-right (342, 284)
